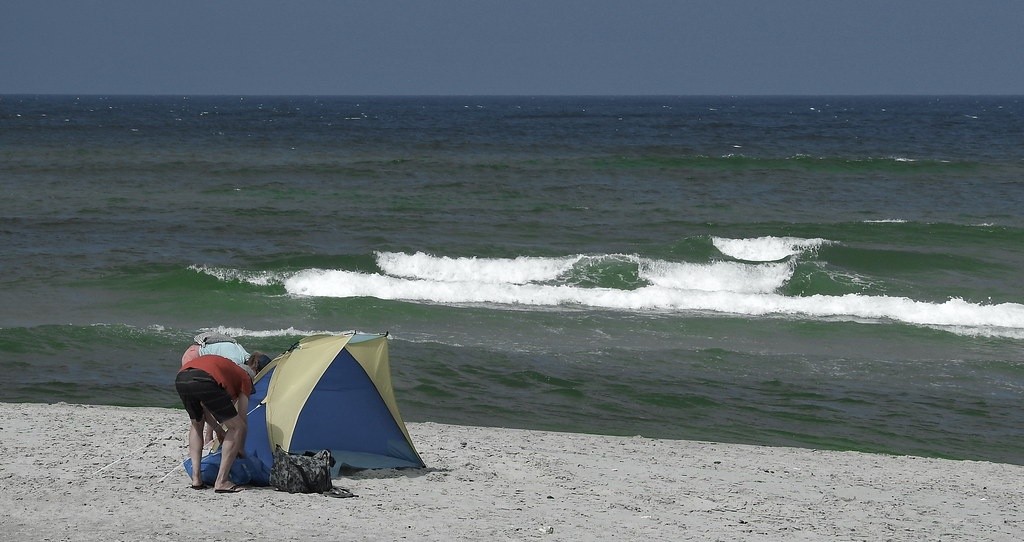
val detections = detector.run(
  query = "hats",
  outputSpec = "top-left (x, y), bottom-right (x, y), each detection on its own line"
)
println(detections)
top-left (254, 352), bottom-right (272, 372)
top-left (239, 363), bottom-right (256, 394)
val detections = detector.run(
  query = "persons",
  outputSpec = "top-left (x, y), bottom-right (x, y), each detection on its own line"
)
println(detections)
top-left (181, 333), bottom-right (274, 447)
top-left (176, 355), bottom-right (258, 495)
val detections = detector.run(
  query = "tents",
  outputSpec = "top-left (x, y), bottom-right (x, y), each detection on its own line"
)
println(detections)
top-left (182, 329), bottom-right (428, 489)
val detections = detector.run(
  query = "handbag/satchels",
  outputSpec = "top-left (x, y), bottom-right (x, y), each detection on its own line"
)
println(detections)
top-left (193, 331), bottom-right (238, 348)
top-left (183, 449), bottom-right (268, 489)
top-left (269, 443), bottom-right (337, 493)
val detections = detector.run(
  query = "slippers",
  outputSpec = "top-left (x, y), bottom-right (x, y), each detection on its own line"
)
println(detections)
top-left (190, 482), bottom-right (207, 489)
top-left (215, 484), bottom-right (246, 493)
